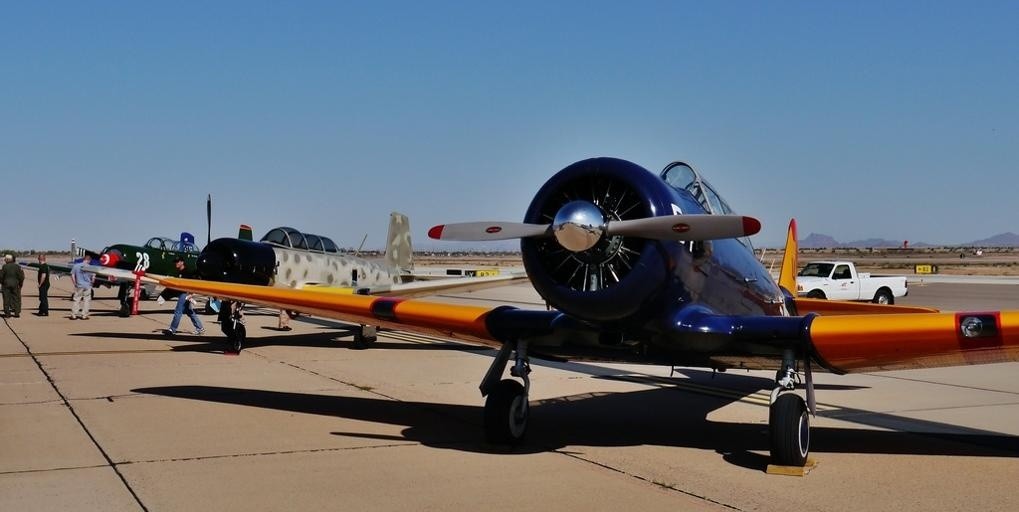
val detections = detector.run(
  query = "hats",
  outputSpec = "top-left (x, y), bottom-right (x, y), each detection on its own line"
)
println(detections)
top-left (172, 256), bottom-right (184, 263)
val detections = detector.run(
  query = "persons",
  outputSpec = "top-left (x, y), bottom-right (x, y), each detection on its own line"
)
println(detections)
top-left (69, 255), bottom-right (97, 320)
top-left (0, 255), bottom-right (23, 317)
top-left (161, 256), bottom-right (207, 335)
top-left (31, 254), bottom-right (51, 316)
top-left (278, 308), bottom-right (293, 332)
top-left (9, 256), bottom-right (25, 313)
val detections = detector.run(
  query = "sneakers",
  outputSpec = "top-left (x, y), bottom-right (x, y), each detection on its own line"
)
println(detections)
top-left (33, 313), bottom-right (49, 316)
top-left (71, 316), bottom-right (90, 320)
top-left (279, 326), bottom-right (291, 331)
top-left (163, 329), bottom-right (174, 335)
top-left (191, 326), bottom-right (205, 334)
top-left (4, 314), bottom-right (20, 318)
